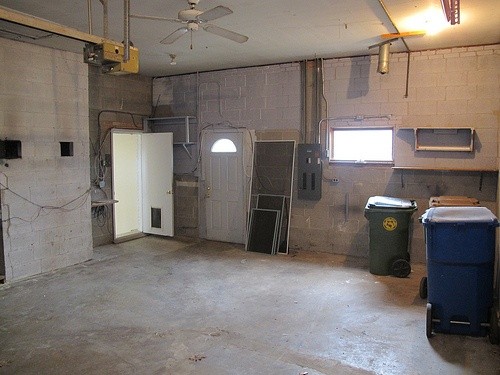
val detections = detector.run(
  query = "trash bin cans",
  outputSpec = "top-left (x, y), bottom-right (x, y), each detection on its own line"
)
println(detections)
top-left (365, 195), bottom-right (420, 279)
top-left (418, 204), bottom-right (500, 339)
top-left (428, 195), bottom-right (479, 208)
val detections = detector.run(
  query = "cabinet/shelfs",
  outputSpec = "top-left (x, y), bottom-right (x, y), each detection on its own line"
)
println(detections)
top-left (391, 127), bottom-right (500, 191)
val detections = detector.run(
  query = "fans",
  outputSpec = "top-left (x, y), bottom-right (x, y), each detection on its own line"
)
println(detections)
top-left (129, 0), bottom-right (248, 46)
